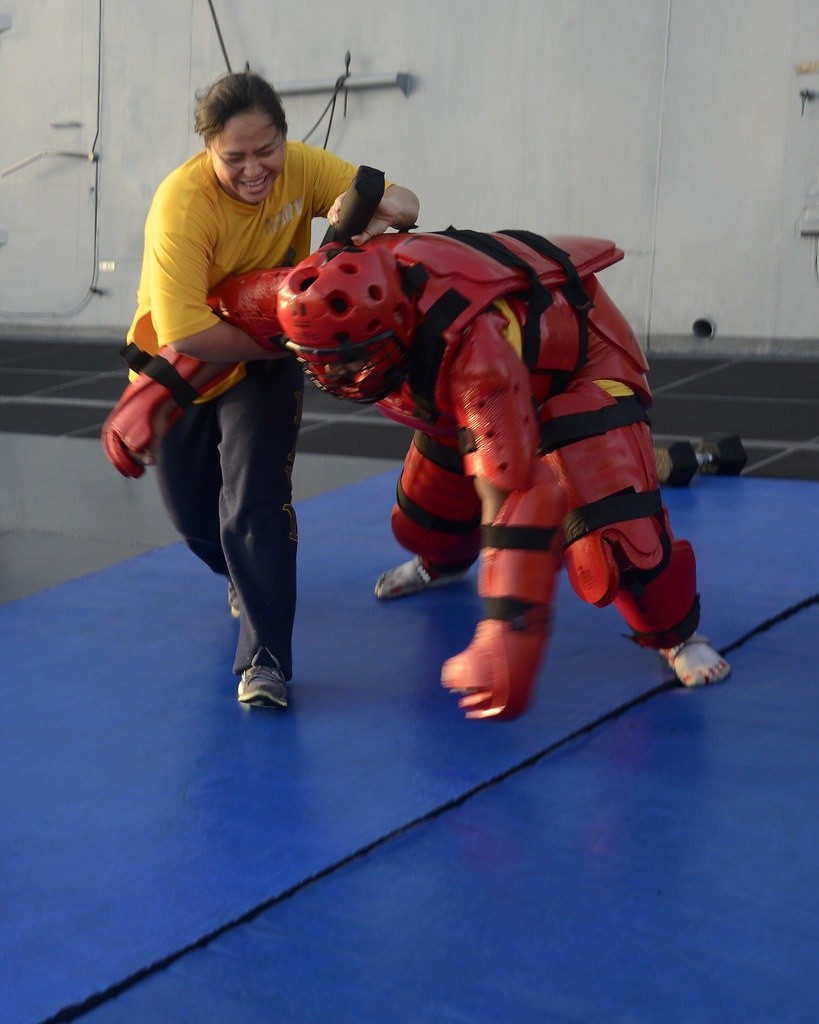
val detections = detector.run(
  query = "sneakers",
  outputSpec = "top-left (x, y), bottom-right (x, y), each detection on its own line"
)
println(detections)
top-left (236, 658), bottom-right (290, 713)
top-left (225, 578), bottom-right (245, 621)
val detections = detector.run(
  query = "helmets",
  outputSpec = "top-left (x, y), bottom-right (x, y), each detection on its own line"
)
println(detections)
top-left (273, 246), bottom-right (395, 345)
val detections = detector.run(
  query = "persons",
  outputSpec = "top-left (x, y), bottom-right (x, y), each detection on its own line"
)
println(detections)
top-left (126, 71), bottom-right (419, 711)
top-left (103, 229), bottom-right (732, 723)
